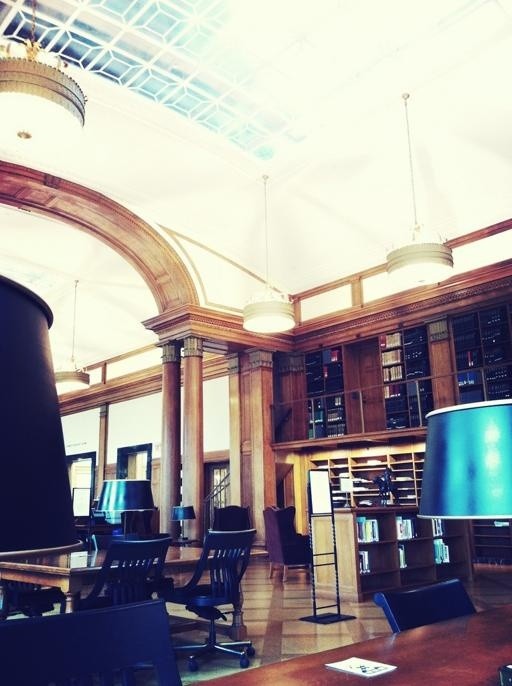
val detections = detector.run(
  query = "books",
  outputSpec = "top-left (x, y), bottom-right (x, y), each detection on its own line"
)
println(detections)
top-left (380, 325), bottom-right (434, 429)
top-left (395, 515), bottom-right (418, 541)
top-left (449, 302), bottom-right (512, 404)
top-left (306, 350), bottom-right (348, 439)
top-left (432, 518), bottom-right (443, 538)
top-left (434, 539), bottom-right (450, 564)
top-left (359, 550), bottom-right (370, 574)
top-left (399, 544), bottom-right (408, 570)
top-left (324, 656), bottom-right (399, 679)
top-left (355, 516), bottom-right (379, 543)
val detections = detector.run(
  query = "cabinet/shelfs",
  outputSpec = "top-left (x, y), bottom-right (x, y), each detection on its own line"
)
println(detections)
top-left (308, 343), bottom-right (347, 441)
top-left (308, 447), bottom-right (512, 603)
top-left (379, 321), bottom-right (435, 433)
top-left (453, 298), bottom-right (512, 407)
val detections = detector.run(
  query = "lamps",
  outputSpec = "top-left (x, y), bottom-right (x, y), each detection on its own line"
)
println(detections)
top-left (0, 0), bottom-right (89, 144)
top-left (96, 479), bottom-right (155, 540)
top-left (169, 507), bottom-right (196, 541)
top-left (241, 91), bottom-right (296, 336)
top-left (54, 279), bottom-right (92, 392)
top-left (383, 91), bottom-right (454, 287)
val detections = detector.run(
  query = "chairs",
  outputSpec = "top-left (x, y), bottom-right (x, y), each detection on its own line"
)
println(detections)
top-left (262, 505), bottom-right (313, 583)
top-left (5, 594), bottom-right (181, 685)
top-left (373, 578), bottom-right (478, 633)
top-left (3, 579), bottom-right (68, 620)
top-left (166, 525), bottom-right (262, 673)
top-left (72, 535), bottom-right (172, 614)
top-left (120, 508), bottom-right (160, 541)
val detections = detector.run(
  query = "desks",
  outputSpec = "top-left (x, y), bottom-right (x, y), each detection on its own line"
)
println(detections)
top-left (184, 602), bottom-right (512, 686)
top-left (0, 539), bottom-right (269, 626)
